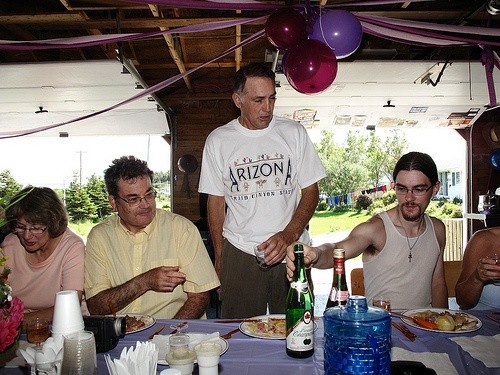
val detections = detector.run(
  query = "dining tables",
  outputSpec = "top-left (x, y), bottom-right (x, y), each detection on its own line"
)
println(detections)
top-left (0, 308), bottom-right (500, 375)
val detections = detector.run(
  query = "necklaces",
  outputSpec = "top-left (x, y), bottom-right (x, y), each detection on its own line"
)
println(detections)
top-left (398, 205), bottom-right (424, 262)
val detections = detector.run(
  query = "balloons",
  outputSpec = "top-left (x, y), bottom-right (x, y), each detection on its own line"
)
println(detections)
top-left (309, 10), bottom-right (362, 59)
top-left (265, 7), bottom-right (307, 50)
top-left (285, 39), bottom-right (338, 94)
top-left (281, 47), bottom-right (319, 81)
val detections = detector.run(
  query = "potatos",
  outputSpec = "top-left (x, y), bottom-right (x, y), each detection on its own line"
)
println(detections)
top-left (434, 315), bottom-right (456, 330)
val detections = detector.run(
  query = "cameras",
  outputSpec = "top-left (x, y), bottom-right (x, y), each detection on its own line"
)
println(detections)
top-left (81, 314), bottom-right (127, 354)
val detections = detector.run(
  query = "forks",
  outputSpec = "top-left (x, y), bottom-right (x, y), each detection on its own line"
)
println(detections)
top-left (221, 329), bottom-right (239, 340)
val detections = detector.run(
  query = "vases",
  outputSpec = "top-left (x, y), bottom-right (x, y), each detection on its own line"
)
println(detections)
top-left (0, 327), bottom-right (22, 368)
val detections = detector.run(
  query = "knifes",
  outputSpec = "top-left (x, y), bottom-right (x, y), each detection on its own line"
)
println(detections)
top-left (148, 325), bottom-right (165, 339)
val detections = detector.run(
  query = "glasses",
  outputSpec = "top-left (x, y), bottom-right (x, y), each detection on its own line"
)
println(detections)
top-left (118, 188), bottom-right (157, 207)
top-left (393, 185), bottom-right (433, 195)
top-left (14, 221), bottom-right (47, 234)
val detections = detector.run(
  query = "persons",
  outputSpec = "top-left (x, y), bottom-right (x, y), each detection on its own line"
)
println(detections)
top-left (455, 227), bottom-right (500, 310)
top-left (84, 155), bottom-right (220, 319)
top-left (198, 61), bottom-right (328, 319)
top-left (0, 187), bottom-right (90, 328)
top-left (286, 152), bottom-right (450, 308)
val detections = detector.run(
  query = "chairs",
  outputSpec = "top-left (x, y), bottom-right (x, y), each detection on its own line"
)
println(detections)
top-left (351, 267), bottom-right (365, 297)
top-left (444, 260), bottom-right (462, 298)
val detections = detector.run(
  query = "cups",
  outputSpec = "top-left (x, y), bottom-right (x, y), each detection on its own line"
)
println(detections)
top-left (373, 295), bottom-right (391, 311)
top-left (255, 246), bottom-right (268, 271)
top-left (50, 290), bottom-right (96, 375)
top-left (486, 253), bottom-right (500, 267)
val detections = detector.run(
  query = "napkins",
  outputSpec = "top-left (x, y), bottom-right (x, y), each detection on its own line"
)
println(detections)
top-left (152, 331), bottom-right (219, 363)
top-left (390, 347), bottom-right (459, 375)
top-left (447, 334), bottom-right (500, 368)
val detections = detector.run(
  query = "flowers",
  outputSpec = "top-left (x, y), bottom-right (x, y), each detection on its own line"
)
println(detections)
top-left (0, 246), bottom-right (25, 353)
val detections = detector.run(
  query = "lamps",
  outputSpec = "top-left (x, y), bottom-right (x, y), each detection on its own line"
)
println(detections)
top-left (383, 100), bottom-right (396, 114)
top-left (147, 97), bottom-right (155, 101)
top-left (134, 81), bottom-right (144, 89)
top-left (121, 65), bottom-right (130, 74)
top-left (114, 45), bottom-right (121, 55)
top-left (35, 106), bottom-right (48, 119)
top-left (115, 55), bottom-right (123, 64)
top-left (155, 104), bottom-right (164, 112)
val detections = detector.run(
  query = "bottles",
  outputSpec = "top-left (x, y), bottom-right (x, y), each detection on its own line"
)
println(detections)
top-left (325, 249), bottom-right (350, 310)
top-left (324, 295), bottom-right (392, 375)
top-left (160, 323), bottom-right (222, 375)
top-left (286, 244), bottom-right (315, 359)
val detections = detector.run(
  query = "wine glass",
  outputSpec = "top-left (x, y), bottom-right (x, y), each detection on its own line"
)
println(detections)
top-left (28, 320), bottom-right (49, 350)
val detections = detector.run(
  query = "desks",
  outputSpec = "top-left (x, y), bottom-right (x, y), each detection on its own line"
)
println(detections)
top-left (463, 212), bottom-right (500, 228)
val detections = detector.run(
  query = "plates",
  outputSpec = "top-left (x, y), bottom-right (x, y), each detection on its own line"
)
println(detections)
top-left (239, 314), bottom-right (320, 340)
top-left (106, 314), bottom-right (157, 335)
top-left (154, 334), bottom-right (229, 365)
top-left (401, 307), bottom-right (482, 333)
